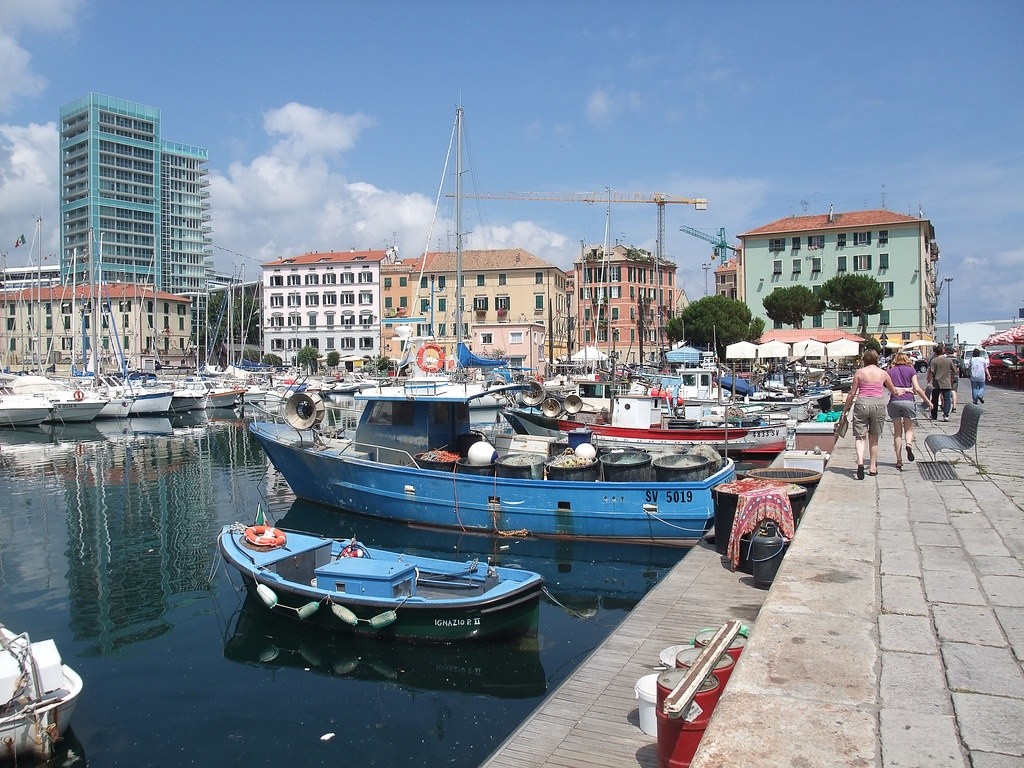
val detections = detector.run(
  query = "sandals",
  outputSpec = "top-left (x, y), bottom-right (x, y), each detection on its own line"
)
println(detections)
top-left (896, 460), bottom-right (903, 468)
top-left (906, 444), bottom-right (914, 462)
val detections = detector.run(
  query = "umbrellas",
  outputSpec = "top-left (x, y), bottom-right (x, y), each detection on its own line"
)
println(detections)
top-left (981, 324), bottom-right (1024, 371)
top-left (714, 374), bottom-right (753, 396)
top-left (317, 350), bottom-right (369, 362)
top-left (564, 344), bottom-right (609, 362)
top-left (902, 339), bottom-right (938, 351)
top-left (877, 340), bottom-right (902, 349)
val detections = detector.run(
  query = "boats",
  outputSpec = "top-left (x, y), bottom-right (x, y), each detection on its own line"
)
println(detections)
top-left (497, 352), bottom-right (853, 458)
top-left (218, 524), bottom-right (545, 647)
top-left (0, 215), bottom-right (393, 425)
top-left (249, 382), bottom-right (737, 549)
top-left (0, 622), bottom-right (84, 762)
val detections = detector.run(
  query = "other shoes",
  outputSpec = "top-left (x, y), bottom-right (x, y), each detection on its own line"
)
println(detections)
top-left (944, 416), bottom-right (949, 422)
top-left (952, 409), bottom-right (956, 413)
top-left (978, 397), bottom-right (984, 403)
top-left (973, 402), bottom-right (977, 404)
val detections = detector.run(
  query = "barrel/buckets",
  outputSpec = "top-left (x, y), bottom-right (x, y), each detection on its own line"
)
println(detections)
top-left (543, 455), bottom-right (599, 481)
top-left (635, 673), bottom-right (659, 738)
top-left (659, 643), bottom-right (694, 671)
top-left (455, 457), bottom-right (496, 477)
top-left (413, 451), bottom-right (460, 473)
top-left (709, 483), bottom-right (806, 556)
top-left (694, 630), bottom-right (750, 664)
top-left (495, 454), bottom-right (545, 481)
top-left (653, 453), bottom-right (710, 483)
top-left (676, 647), bottom-right (734, 694)
top-left (655, 668), bottom-right (720, 768)
top-left (738, 522), bottom-right (792, 588)
top-left (599, 453), bottom-right (653, 483)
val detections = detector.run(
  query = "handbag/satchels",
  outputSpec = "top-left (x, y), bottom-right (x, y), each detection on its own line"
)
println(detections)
top-left (838, 414), bottom-right (849, 438)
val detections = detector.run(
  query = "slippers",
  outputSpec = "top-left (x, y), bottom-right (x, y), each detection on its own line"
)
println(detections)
top-left (857, 465), bottom-right (864, 480)
top-left (870, 471), bottom-right (878, 476)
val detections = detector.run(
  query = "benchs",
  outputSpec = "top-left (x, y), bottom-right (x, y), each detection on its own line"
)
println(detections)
top-left (913, 385), bottom-right (937, 427)
top-left (924, 403), bottom-right (987, 482)
top-left (988, 366), bottom-right (1024, 391)
top-left (482, 578), bottom-right (522, 596)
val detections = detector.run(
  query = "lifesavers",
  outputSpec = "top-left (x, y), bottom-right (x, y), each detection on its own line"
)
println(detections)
top-left (244, 525), bottom-right (287, 547)
top-left (234, 386), bottom-right (239, 390)
top-left (73, 391), bottom-right (85, 401)
top-left (416, 344), bottom-right (445, 373)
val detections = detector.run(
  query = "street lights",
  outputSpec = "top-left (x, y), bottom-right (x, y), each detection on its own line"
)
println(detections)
top-left (945, 277), bottom-right (953, 343)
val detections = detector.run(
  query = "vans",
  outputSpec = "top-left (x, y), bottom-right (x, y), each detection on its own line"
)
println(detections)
top-left (959, 349), bottom-right (990, 378)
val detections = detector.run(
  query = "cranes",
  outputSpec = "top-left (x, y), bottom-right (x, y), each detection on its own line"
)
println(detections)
top-left (679, 226), bottom-right (738, 265)
top-left (443, 193), bottom-right (708, 261)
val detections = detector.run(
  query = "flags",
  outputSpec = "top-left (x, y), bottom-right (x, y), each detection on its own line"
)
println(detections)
top-left (15, 234), bottom-right (26, 248)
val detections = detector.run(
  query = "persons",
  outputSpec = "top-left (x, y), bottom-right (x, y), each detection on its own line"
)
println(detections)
top-left (840, 349), bottom-right (905, 479)
top-left (885, 353), bottom-right (933, 468)
top-left (944, 346), bottom-right (960, 413)
top-left (965, 349), bottom-right (991, 404)
top-left (828, 359), bottom-right (837, 369)
top-left (923, 347), bottom-right (956, 421)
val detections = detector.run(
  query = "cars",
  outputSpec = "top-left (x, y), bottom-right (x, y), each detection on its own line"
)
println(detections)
top-left (903, 350), bottom-right (928, 373)
top-left (989, 351), bottom-right (1024, 365)
